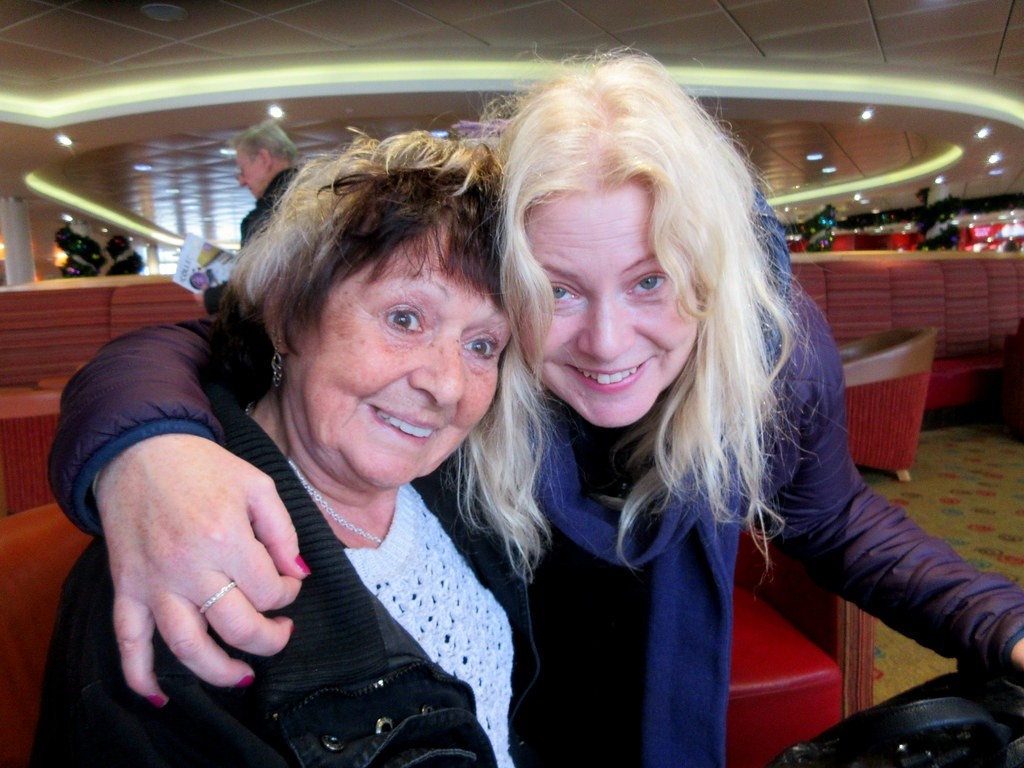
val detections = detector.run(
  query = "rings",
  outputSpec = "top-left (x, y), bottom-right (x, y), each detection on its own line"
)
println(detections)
top-left (200, 581), bottom-right (236, 614)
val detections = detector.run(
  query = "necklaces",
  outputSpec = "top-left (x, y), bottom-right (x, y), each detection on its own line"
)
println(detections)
top-left (288, 460), bottom-right (383, 547)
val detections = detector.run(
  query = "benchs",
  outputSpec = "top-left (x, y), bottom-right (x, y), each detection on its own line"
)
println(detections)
top-left (787, 251), bottom-right (1024, 410)
top-left (0, 274), bottom-right (213, 386)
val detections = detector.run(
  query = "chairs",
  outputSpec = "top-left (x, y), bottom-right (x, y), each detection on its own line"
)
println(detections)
top-left (999, 318), bottom-right (1024, 440)
top-left (836, 324), bottom-right (939, 483)
top-left (0, 498), bottom-right (872, 768)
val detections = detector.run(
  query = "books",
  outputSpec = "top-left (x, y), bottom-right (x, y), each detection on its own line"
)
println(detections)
top-left (173, 232), bottom-right (237, 294)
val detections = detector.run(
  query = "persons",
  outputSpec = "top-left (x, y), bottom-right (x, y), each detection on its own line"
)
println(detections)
top-left (53, 221), bottom-right (144, 278)
top-left (237, 117), bottom-right (303, 249)
top-left (46, 38), bottom-right (1024, 704)
top-left (25, 107), bottom-right (602, 768)
